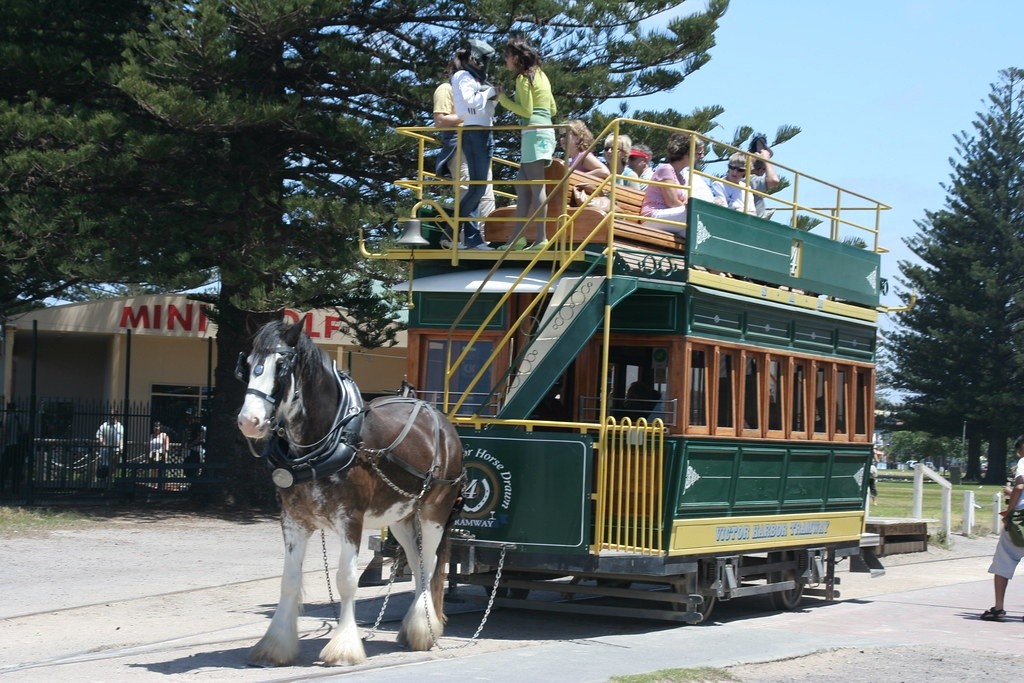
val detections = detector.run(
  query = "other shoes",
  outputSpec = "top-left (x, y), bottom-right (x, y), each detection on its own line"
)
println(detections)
top-left (439, 239), bottom-right (468, 251)
top-left (472, 243), bottom-right (496, 251)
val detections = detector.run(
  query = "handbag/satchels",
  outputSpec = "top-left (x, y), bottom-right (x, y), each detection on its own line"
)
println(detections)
top-left (459, 40), bottom-right (495, 80)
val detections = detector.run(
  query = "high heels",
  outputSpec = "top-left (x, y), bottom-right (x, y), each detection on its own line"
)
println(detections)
top-left (496, 236), bottom-right (527, 251)
top-left (523, 239), bottom-right (549, 251)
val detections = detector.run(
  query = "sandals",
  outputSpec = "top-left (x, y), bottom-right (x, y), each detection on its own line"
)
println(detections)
top-left (981, 605), bottom-right (1007, 621)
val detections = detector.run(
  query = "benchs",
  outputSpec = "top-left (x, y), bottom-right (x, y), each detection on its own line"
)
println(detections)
top-left (483, 157), bottom-right (708, 252)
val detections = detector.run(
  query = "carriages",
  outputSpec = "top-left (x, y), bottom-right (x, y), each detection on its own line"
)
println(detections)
top-left (236, 115), bottom-right (888, 667)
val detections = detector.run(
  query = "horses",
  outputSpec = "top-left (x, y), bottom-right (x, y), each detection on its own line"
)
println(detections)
top-left (235, 306), bottom-right (466, 670)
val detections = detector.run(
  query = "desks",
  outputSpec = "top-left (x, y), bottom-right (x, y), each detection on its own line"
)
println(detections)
top-left (865, 517), bottom-right (939, 556)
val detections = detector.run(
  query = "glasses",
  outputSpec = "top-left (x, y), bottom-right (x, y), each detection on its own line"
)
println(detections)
top-left (727, 163), bottom-right (747, 174)
top-left (695, 150), bottom-right (705, 155)
top-left (602, 146), bottom-right (628, 153)
top-left (629, 155), bottom-right (639, 161)
top-left (558, 132), bottom-right (569, 139)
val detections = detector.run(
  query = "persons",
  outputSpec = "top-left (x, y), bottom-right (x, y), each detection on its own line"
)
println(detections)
top-left (814, 395), bottom-right (846, 434)
top-left (433, 38), bottom-right (559, 252)
top-left (868, 465), bottom-right (878, 506)
top-left (559, 119), bottom-right (655, 198)
top-left (978, 436), bottom-right (1024, 620)
top-left (96, 408), bottom-right (125, 483)
top-left (148, 421), bottom-right (169, 487)
top-left (638, 130), bottom-right (782, 240)
top-left (183, 415), bottom-right (207, 478)
